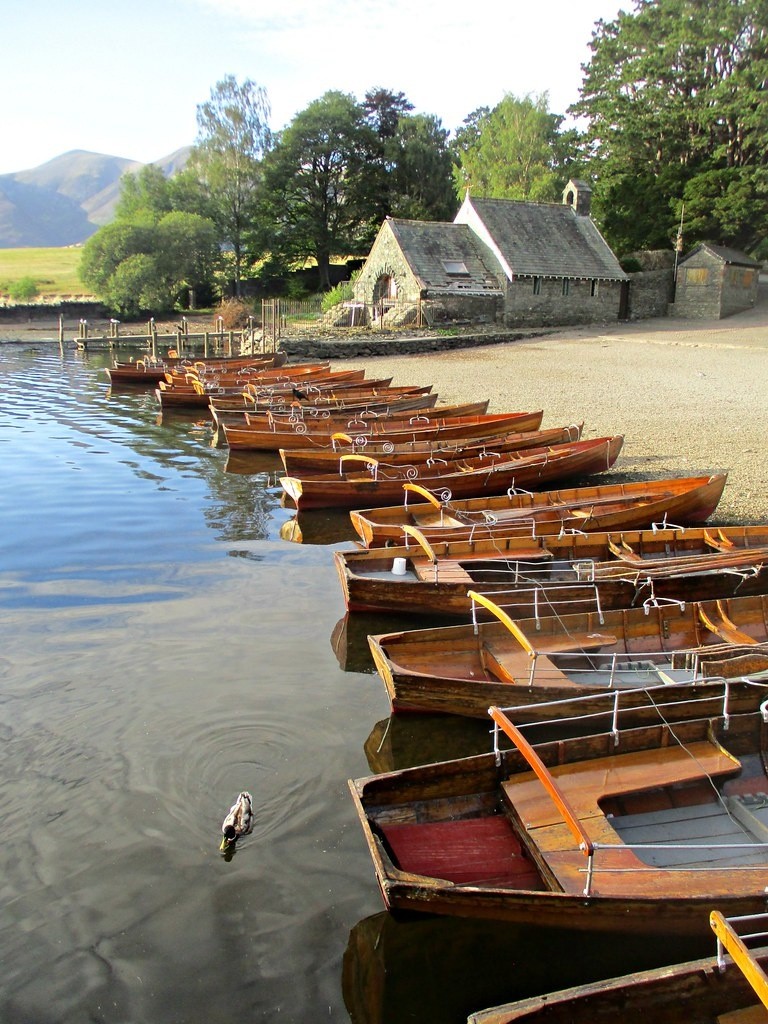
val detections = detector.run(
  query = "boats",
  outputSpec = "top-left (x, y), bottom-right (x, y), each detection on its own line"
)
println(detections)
top-left (275, 432), bottom-right (627, 512)
top-left (332, 524), bottom-right (768, 612)
top-left (281, 507), bottom-right (361, 547)
top-left (345, 676), bottom-right (768, 908)
top-left (100, 353), bottom-right (588, 470)
top-left (222, 450), bottom-right (283, 475)
top-left (154, 407), bottom-right (212, 429)
top-left (369, 580), bottom-right (768, 728)
top-left (358, 716), bottom-right (601, 771)
top-left (336, 909), bottom-right (721, 1024)
top-left (349, 470), bottom-right (735, 549)
top-left (328, 609), bottom-right (461, 677)
top-left (466, 904), bottom-right (768, 1024)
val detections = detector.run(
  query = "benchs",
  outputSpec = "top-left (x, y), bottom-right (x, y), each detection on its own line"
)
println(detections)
top-left (412, 548), bottom-right (768, 893)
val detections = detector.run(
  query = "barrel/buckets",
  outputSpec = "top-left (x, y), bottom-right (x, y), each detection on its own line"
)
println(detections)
top-left (391, 558), bottom-right (407, 575)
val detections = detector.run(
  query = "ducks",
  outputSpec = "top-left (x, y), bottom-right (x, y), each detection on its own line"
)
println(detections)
top-left (220, 791), bottom-right (254, 850)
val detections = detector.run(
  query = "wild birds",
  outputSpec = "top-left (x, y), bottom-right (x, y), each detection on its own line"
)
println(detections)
top-left (80, 318), bottom-right (87, 324)
top-left (219, 316), bottom-right (224, 320)
top-left (151, 317), bottom-right (154, 322)
top-left (111, 318), bottom-right (120, 324)
top-left (182, 316), bottom-right (188, 321)
top-left (291, 388), bottom-right (309, 401)
top-left (177, 325), bottom-right (183, 331)
top-left (249, 315), bottom-right (254, 319)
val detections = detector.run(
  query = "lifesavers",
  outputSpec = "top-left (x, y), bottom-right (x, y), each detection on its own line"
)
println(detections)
top-left (239, 315), bottom-right (250, 328)
